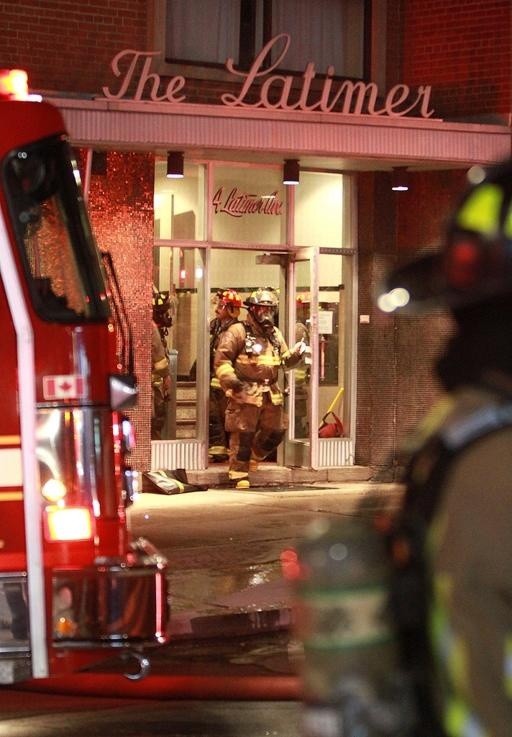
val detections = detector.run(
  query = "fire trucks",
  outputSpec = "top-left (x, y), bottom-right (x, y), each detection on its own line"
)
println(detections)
top-left (0, 64), bottom-right (177, 691)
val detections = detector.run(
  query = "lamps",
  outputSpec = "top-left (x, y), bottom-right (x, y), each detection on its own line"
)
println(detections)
top-left (283, 159), bottom-right (300, 184)
top-left (392, 166), bottom-right (408, 191)
top-left (166, 150), bottom-right (184, 179)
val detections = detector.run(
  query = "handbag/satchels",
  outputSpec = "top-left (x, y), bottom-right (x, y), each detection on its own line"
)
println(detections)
top-left (319, 423), bottom-right (343, 437)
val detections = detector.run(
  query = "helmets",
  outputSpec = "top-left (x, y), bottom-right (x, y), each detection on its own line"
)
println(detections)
top-left (212, 289), bottom-right (241, 307)
top-left (243, 287), bottom-right (280, 307)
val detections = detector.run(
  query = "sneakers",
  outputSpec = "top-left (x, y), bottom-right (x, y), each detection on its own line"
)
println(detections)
top-left (208, 454), bottom-right (229, 464)
top-left (230, 476), bottom-right (249, 489)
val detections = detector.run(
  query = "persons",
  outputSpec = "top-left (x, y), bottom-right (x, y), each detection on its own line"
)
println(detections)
top-left (151, 281), bottom-right (324, 490)
top-left (353, 161), bottom-right (511, 737)
